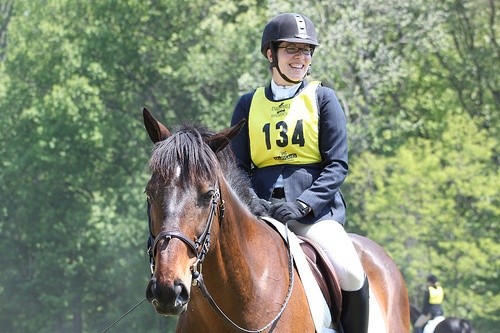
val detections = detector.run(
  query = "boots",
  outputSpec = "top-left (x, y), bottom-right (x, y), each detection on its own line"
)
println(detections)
top-left (341, 277), bottom-right (369, 333)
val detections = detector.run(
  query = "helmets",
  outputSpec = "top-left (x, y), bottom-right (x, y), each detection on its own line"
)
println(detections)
top-left (261, 13), bottom-right (319, 59)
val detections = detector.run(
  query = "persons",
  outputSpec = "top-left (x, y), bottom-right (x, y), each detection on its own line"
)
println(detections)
top-left (231, 12), bottom-right (370, 333)
top-left (414, 275), bottom-right (444, 333)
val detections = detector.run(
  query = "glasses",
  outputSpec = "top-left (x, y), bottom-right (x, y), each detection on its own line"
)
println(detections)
top-left (278, 44), bottom-right (312, 55)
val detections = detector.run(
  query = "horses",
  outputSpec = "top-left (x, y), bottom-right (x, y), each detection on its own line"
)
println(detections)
top-left (410, 307), bottom-right (471, 333)
top-left (143, 108), bottom-right (412, 333)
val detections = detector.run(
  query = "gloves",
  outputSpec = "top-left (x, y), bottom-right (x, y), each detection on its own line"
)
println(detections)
top-left (259, 199), bottom-right (271, 212)
top-left (268, 202), bottom-right (309, 225)
